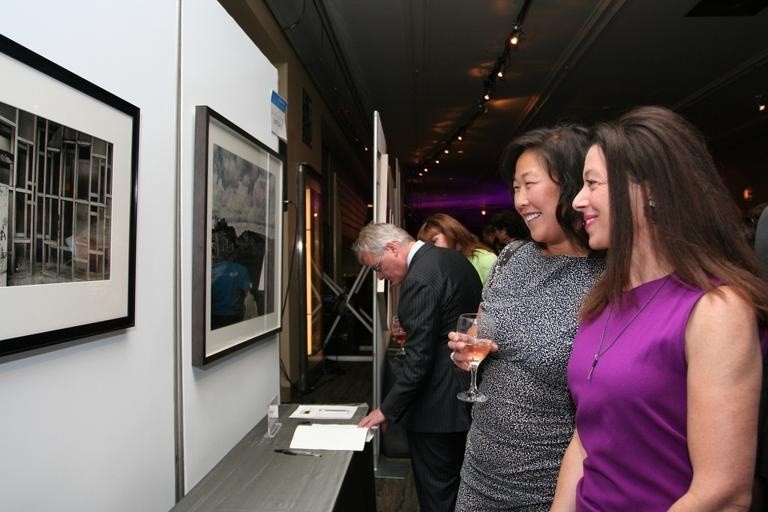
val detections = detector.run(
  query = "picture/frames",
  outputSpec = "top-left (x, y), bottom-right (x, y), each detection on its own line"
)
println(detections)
top-left (191, 104), bottom-right (285, 369)
top-left (0, 34), bottom-right (141, 359)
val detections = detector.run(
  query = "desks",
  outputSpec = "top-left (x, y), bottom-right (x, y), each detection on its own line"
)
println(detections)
top-left (168, 401), bottom-right (376, 512)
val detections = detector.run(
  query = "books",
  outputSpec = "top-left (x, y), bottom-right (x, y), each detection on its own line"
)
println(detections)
top-left (288, 404), bottom-right (358, 420)
top-left (290, 422), bottom-right (377, 452)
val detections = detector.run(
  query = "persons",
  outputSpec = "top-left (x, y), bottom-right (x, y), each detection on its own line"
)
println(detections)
top-left (447, 123), bottom-right (608, 512)
top-left (549, 104), bottom-right (768, 512)
top-left (416, 213), bottom-right (498, 287)
top-left (351, 221), bottom-right (484, 511)
top-left (479, 226), bottom-right (506, 256)
top-left (490, 209), bottom-right (529, 246)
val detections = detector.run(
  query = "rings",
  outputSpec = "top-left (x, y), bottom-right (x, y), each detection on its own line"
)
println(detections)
top-left (449, 351), bottom-right (455, 361)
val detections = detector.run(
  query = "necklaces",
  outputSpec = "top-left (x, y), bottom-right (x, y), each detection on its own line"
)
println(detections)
top-left (585, 272), bottom-right (673, 382)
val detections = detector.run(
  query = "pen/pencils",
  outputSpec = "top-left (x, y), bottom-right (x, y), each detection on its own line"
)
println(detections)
top-left (275, 449), bottom-right (322, 457)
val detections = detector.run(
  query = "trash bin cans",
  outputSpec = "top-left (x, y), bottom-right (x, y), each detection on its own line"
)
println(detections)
top-left (322, 300), bottom-right (359, 355)
top-left (381, 377), bottom-right (409, 458)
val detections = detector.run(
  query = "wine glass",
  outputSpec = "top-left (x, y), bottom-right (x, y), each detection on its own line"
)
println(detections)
top-left (392, 315), bottom-right (409, 356)
top-left (456, 313), bottom-right (493, 403)
